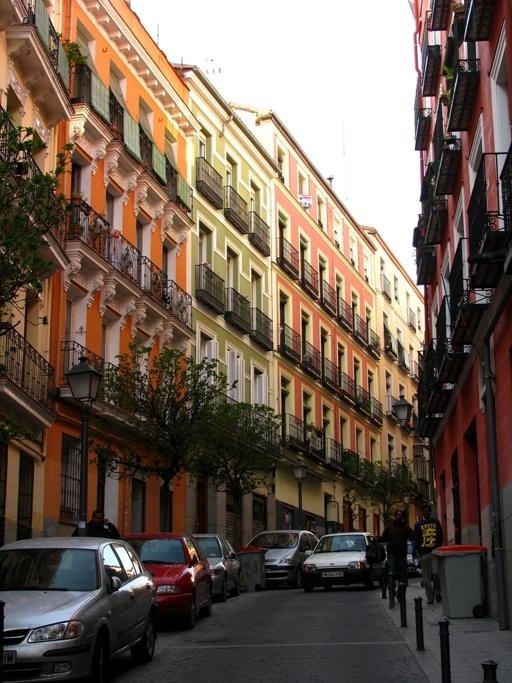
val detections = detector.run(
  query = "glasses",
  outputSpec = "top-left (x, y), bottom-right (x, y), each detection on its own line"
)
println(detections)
top-left (366, 537), bottom-right (385, 562)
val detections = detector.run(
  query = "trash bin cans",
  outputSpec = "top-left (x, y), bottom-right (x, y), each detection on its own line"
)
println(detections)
top-left (431, 544), bottom-right (488, 619)
top-left (238, 546), bottom-right (268, 593)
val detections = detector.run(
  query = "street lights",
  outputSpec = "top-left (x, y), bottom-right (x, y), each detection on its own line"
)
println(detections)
top-left (402, 487), bottom-right (412, 522)
top-left (63, 354), bottom-right (102, 539)
top-left (287, 449), bottom-right (309, 529)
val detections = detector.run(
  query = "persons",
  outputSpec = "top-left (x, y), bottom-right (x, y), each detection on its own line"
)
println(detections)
top-left (373, 510), bottom-right (414, 585)
top-left (412, 506), bottom-right (443, 604)
top-left (72, 509), bottom-right (121, 540)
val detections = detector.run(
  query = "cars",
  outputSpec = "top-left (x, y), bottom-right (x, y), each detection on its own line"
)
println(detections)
top-left (192, 533), bottom-right (244, 602)
top-left (406, 541), bottom-right (418, 575)
top-left (301, 529), bottom-right (386, 593)
top-left (0, 537), bottom-right (160, 680)
top-left (245, 529), bottom-right (322, 589)
top-left (122, 531), bottom-right (214, 629)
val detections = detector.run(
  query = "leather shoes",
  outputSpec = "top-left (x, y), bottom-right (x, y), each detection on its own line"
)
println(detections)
top-left (436, 594), bottom-right (441, 601)
top-left (427, 596), bottom-right (434, 604)
